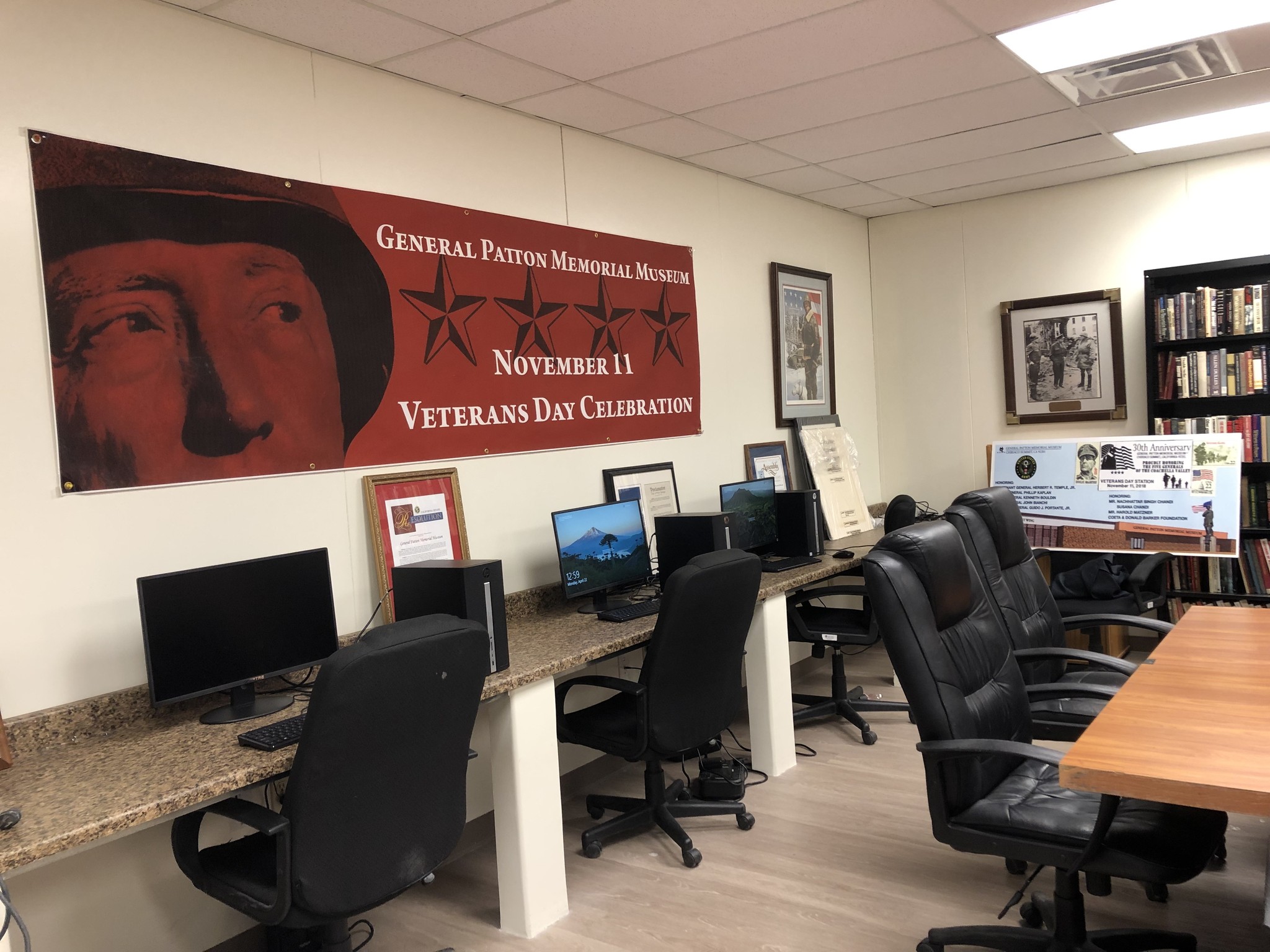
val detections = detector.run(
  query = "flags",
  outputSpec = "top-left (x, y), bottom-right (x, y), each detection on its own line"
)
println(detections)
top-left (1192, 500), bottom-right (1211, 513)
top-left (1193, 469), bottom-right (1214, 481)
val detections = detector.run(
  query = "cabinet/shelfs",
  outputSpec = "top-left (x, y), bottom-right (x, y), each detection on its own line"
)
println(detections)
top-left (1143, 254), bottom-right (1270, 607)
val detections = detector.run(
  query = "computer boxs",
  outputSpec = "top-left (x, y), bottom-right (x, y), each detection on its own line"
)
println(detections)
top-left (391, 559), bottom-right (509, 676)
top-left (654, 511), bottom-right (738, 592)
top-left (775, 489), bottom-right (824, 558)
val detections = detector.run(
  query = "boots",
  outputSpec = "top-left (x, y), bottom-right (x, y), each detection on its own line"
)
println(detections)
top-left (1087, 375), bottom-right (1092, 390)
top-left (1030, 385), bottom-right (1043, 400)
top-left (1078, 373), bottom-right (1085, 386)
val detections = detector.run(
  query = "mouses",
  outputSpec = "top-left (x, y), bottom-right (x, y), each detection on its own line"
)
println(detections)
top-left (832, 550), bottom-right (854, 558)
top-left (0, 810), bottom-right (21, 830)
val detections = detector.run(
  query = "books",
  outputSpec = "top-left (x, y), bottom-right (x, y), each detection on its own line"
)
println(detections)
top-left (1166, 537), bottom-right (1270, 595)
top-left (1153, 280), bottom-right (1270, 341)
top-left (1240, 476), bottom-right (1270, 528)
top-left (1154, 413), bottom-right (1270, 463)
top-left (1166, 598), bottom-right (1270, 627)
top-left (1158, 343), bottom-right (1270, 400)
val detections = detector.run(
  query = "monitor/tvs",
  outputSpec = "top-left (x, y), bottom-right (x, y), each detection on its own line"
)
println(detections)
top-left (552, 498), bottom-right (652, 615)
top-left (719, 476), bottom-right (779, 560)
top-left (137, 547), bottom-right (340, 726)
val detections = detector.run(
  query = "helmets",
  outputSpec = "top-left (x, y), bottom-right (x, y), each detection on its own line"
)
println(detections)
top-left (1030, 333), bottom-right (1039, 341)
top-left (1080, 332), bottom-right (1088, 338)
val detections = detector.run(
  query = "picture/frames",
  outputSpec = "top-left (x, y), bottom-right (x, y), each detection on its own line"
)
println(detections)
top-left (769, 260), bottom-right (837, 430)
top-left (361, 466), bottom-right (472, 626)
top-left (792, 413), bottom-right (842, 488)
top-left (601, 461), bottom-right (682, 516)
top-left (742, 440), bottom-right (795, 496)
top-left (999, 286), bottom-right (1130, 427)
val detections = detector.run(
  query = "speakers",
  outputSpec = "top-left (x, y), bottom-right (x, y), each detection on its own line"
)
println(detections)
top-left (884, 495), bottom-right (915, 535)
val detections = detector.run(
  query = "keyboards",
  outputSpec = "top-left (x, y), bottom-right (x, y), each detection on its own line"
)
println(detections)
top-left (597, 598), bottom-right (663, 623)
top-left (237, 711), bottom-right (310, 751)
top-left (762, 556), bottom-right (822, 573)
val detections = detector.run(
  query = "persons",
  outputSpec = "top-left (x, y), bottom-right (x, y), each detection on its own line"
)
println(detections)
top-left (1075, 333), bottom-right (1096, 390)
top-left (1025, 333), bottom-right (1042, 401)
top-left (1048, 333), bottom-right (1067, 389)
top-left (1101, 446), bottom-right (1116, 470)
top-left (1164, 472), bottom-right (1188, 489)
top-left (1077, 443), bottom-right (1098, 481)
top-left (802, 296), bottom-right (821, 400)
top-left (1201, 505), bottom-right (1214, 536)
top-left (32, 131), bottom-right (397, 493)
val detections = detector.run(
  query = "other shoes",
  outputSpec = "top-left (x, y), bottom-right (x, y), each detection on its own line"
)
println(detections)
top-left (1054, 385), bottom-right (1058, 389)
top-left (1059, 384), bottom-right (1065, 388)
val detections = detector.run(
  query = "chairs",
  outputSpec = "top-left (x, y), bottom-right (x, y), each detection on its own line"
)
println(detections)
top-left (553, 547), bottom-right (763, 871)
top-left (785, 494), bottom-right (923, 746)
top-left (170, 614), bottom-right (491, 952)
top-left (861, 484), bottom-right (1230, 952)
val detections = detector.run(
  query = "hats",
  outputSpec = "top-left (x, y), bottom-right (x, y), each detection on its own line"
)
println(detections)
top-left (1077, 444), bottom-right (1098, 461)
top-left (30, 129), bottom-right (396, 450)
top-left (1056, 334), bottom-right (1065, 339)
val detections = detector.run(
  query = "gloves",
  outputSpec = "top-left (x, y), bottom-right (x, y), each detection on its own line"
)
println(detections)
top-left (1087, 358), bottom-right (1094, 367)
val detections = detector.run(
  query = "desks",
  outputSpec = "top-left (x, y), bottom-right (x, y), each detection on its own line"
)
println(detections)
top-left (1055, 604), bottom-right (1270, 820)
top-left (0, 524), bottom-right (887, 886)
